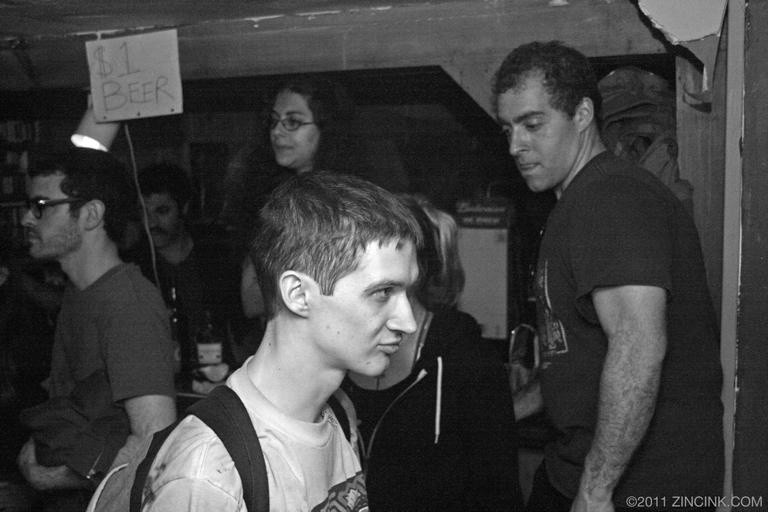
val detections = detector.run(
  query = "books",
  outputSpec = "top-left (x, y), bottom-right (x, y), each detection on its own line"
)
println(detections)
top-left (1, 117), bottom-right (51, 251)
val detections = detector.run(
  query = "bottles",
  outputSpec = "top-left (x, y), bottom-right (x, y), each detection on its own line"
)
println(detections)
top-left (194, 310), bottom-right (224, 365)
top-left (167, 286), bottom-right (192, 374)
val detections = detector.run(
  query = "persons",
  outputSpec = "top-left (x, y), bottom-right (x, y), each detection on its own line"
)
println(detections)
top-left (489, 38), bottom-right (729, 512)
top-left (116, 162), bottom-right (264, 418)
top-left (227, 71), bottom-right (364, 263)
top-left (325, 192), bottom-right (526, 511)
top-left (124, 166), bottom-right (431, 512)
top-left (1, 141), bottom-right (181, 511)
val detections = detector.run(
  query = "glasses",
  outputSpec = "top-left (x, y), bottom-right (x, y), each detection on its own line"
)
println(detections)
top-left (25, 195), bottom-right (82, 219)
top-left (261, 114), bottom-right (317, 131)
